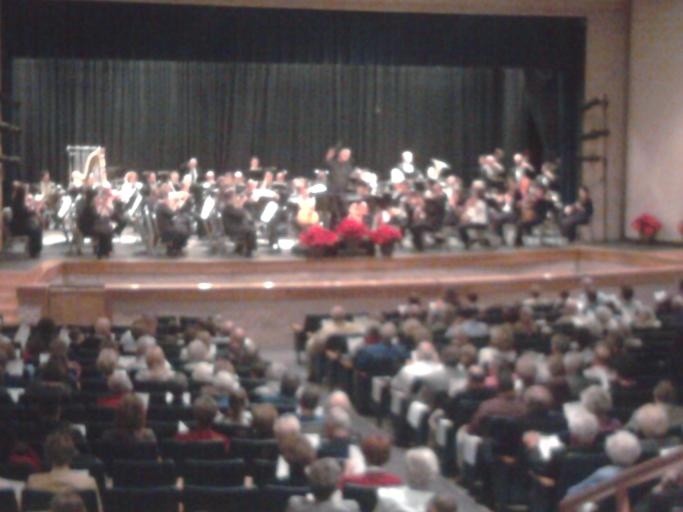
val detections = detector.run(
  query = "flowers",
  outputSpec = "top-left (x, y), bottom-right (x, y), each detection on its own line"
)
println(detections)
top-left (300, 219), bottom-right (403, 258)
top-left (631, 213), bottom-right (662, 239)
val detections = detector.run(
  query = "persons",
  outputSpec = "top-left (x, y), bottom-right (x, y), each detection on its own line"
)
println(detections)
top-left (8, 139), bottom-right (594, 258)
top-left (1, 280), bottom-right (682, 511)
